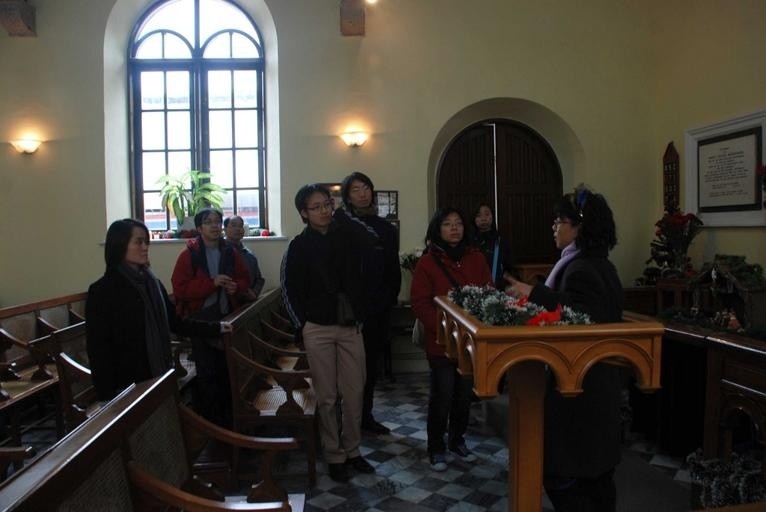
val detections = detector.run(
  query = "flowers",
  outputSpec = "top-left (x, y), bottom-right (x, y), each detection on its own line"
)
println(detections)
top-left (644, 213), bottom-right (703, 274)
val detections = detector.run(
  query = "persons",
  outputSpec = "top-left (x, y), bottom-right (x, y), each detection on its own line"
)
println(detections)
top-left (465, 202), bottom-right (514, 289)
top-left (224, 216), bottom-right (265, 302)
top-left (280, 185), bottom-right (380, 482)
top-left (171, 208), bottom-right (251, 430)
top-left (502, 185), bottom-right (625, 512)
top-left (84, 218), bottom-right (233, 409)
top-left (341, 172), bottom-right (401, 435)
top-left (410, 206), bottom-right (493, 472)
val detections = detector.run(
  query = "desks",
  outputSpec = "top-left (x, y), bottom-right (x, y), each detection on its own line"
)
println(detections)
top-left (621, 311), bottom-right (766, 482)
top-left (384, 305), bottom-right (418, 384)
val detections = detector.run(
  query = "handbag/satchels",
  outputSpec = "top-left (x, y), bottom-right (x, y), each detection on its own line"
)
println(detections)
top-left (187, 303), bottom-right (223, 339)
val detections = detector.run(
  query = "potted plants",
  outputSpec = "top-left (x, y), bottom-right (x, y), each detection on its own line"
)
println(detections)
top-left (155, 171), bottom-right (227, 234)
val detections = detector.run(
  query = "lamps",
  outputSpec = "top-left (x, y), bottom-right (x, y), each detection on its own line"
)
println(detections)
top-left (341, 124), bottom-right (369, 147)
top-left (7, 131), bottom-right (43, 154)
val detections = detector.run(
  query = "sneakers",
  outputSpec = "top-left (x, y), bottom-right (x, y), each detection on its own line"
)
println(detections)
top-left (449, 441), bottom-right (479, 463)
top-left (428, 450), bottom-right (449, 472)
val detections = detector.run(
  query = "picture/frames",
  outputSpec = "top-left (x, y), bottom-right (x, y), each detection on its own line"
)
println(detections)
top-left (681, 111), bottom-right (766, 229)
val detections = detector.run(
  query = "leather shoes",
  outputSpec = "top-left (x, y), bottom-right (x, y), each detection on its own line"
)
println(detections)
top-left (345, 454), bottom-right (375, 473)
top-left (363, 414), bottom-right (391, 436)
top-left (327, 459), bottom-right (350, 484)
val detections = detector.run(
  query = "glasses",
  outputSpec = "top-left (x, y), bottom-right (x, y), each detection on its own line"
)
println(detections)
top-left (552, 218), bottom-right (574, 227)
top-left (304, 200), bottom-right (333, 214)
